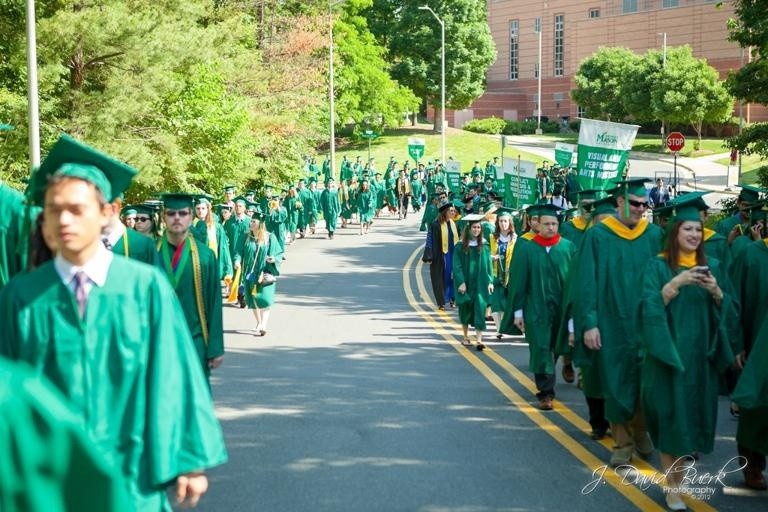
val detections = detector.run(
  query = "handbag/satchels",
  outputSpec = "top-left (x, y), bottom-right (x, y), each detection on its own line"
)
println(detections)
top-left (261, 273), bottom-right (277, 286)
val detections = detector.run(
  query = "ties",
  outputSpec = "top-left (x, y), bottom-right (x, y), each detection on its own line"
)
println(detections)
top-left (74, 272), bottom-right (88, 318)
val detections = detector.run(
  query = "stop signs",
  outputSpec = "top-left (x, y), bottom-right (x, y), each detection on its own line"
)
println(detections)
top-left (666, 132), bottom-right (685, 151)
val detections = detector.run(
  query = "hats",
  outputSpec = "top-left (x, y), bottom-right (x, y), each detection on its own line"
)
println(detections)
top-left (14, 135), bottom-right (138, 256)
top-left (741, 182), bottom-right (768, 233)
top-left (431, 171), bottom-right (709, 241)
top-left (120, 177), bottom-right (335, 233)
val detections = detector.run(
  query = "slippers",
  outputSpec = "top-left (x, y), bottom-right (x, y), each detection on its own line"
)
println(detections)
top-left (477, 342), bottom-right (485, 351)
top-left (462, 339), bottom-right (472, 346)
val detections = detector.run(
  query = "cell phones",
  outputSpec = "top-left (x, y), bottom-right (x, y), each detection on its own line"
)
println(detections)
top-left (694, 266), bottom-right (709, 284)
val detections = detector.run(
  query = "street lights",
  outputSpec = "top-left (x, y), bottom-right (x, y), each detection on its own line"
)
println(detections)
top-left (418, 4), bottom-right (447, 169)
top-left (532, 29), bottom-right (544, 136)
top-left (327, 0), bottom-right (355, 181)
top-left (657, 31), bottom-right (667, 149)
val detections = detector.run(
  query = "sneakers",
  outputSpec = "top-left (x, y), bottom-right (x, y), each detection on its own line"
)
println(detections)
top-left (610, 448), bottom-right (631, 468)
top-left (449, 298), bottom-right (456, 308)
top-left (633, 433), bottom-right (653, 454)
top-left (439, 306), bottom-right (444, 310)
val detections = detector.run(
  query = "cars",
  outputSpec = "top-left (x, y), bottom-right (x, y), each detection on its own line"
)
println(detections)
top-left (522, 116), bottom-right (548, 124)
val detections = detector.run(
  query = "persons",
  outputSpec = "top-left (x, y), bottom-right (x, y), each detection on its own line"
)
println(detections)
top-left (638, 184), bottom-right (737, 511)
top-left (1, 155), bottom-right (768, 490)
top-left (1, 355), bottom-right (135, 512)
top-left (0, 135), bottom-right (225, 512)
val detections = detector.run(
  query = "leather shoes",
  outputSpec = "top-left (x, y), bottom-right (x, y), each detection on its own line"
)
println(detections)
top-left (562, 363), bottom-right (575, 382)
top-left (539, 398), bottom-right (553, 409)
top-left (591, 428), bottom-right (608, 440)
top-left (740, 463), bottom-right (767, 490)
top-left (665, 493), bottom-right (686, 510)
